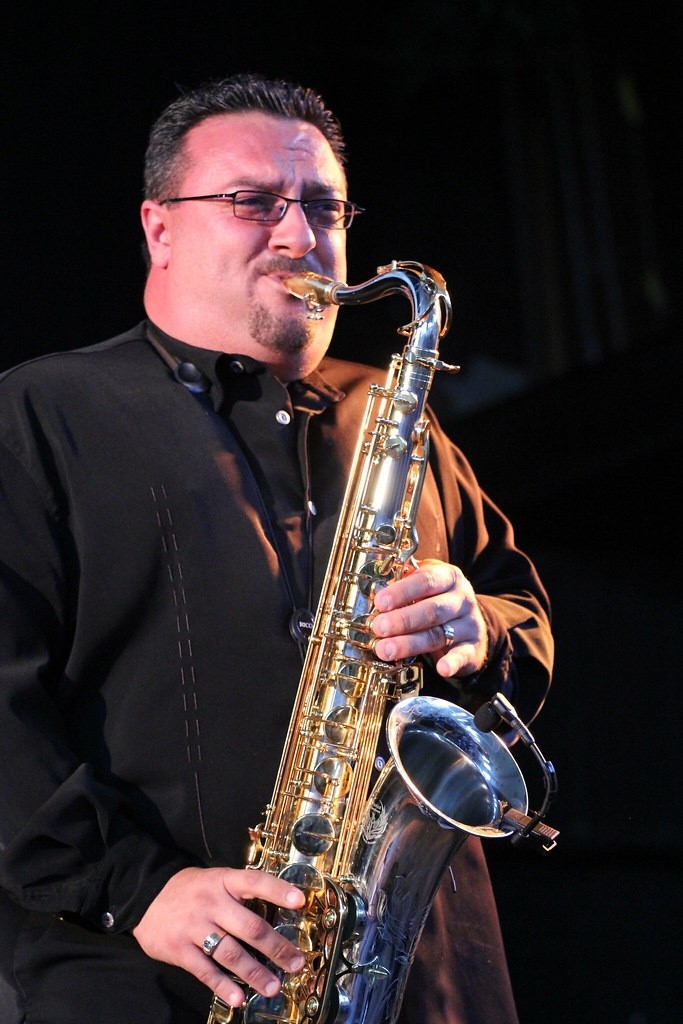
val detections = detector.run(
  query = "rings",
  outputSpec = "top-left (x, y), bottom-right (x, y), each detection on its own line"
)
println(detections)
top-left (442, 623), bottom-right (455, 647)
top-left (202, 930), bottom-right (228, 956)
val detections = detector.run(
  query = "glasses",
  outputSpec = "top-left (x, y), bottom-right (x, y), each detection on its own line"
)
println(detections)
top-left (159, 190), bottom-right (364, 230)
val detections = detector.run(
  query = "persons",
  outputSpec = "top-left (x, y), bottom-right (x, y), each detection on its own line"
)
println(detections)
top-left (1, 74), bottom-right (554, 1024)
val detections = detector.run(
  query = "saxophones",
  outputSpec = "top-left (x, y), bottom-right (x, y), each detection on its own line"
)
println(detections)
top-left (204, 257), bottom-right (533, 1023)
